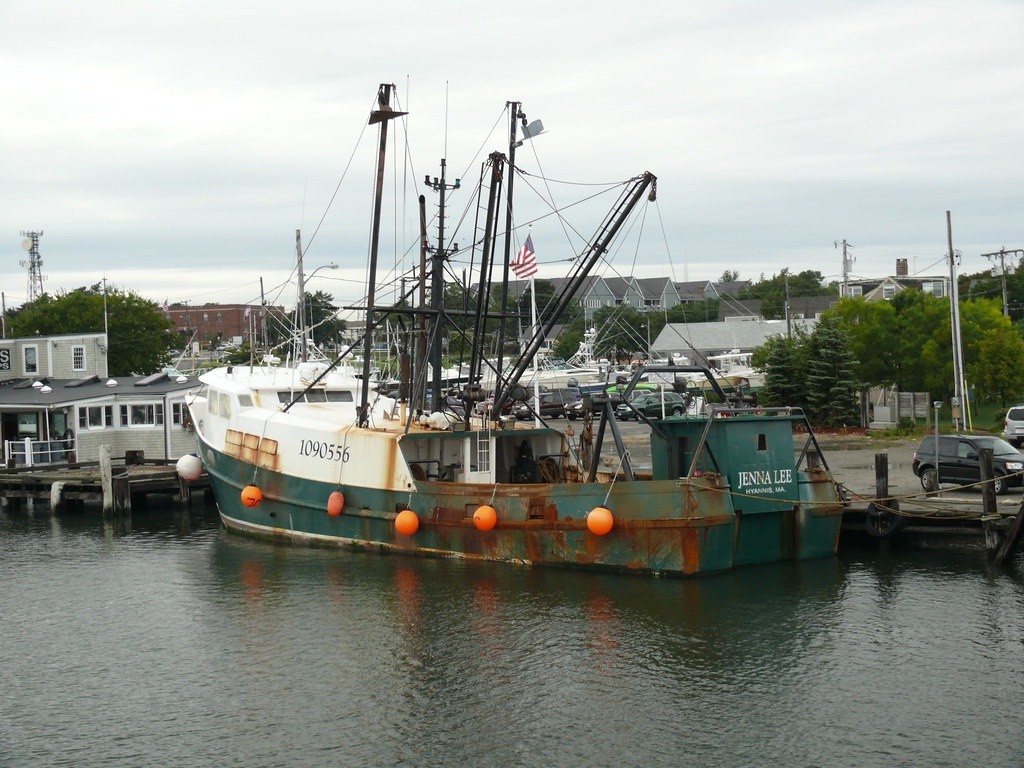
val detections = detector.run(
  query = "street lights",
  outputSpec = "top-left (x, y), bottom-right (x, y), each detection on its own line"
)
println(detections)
top-left (298, 262), bottom-right (338, 363)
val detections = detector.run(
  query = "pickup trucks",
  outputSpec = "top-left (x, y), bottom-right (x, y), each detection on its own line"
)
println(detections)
top-left (616, 390), bottom-right (686, 421)
top-left (912, 435), bottom-right (1024, 493)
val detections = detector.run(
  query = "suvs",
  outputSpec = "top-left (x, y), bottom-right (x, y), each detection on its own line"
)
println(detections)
top-left (563, 393), bottom-right (623, 420)
top-left (515, 392), bottom-right (575, 419)
top-left (1001, 406), bottom-right (1024, 448)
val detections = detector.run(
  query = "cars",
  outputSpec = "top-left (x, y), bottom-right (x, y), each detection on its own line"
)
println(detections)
top-left (476, 395), bottom-right (514, 416)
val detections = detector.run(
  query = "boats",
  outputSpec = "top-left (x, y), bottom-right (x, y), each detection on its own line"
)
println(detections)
top-left (186, 75), bottom-right (846, 577)
top-left (642, 318), bottom-right (767, 392)
top-left (339, 300), bottom-right (639, 391)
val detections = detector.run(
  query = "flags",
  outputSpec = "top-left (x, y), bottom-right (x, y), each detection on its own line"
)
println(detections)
top-left (162, 300), bottom-right (168, 312)
top-left (245, 306), bottom-right (250, 317)
top-left (510, 234), bottom-right (538, 279)
